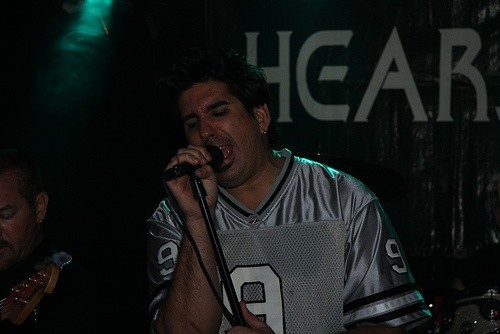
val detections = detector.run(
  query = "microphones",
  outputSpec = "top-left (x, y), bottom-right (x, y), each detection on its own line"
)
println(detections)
top-left (160, 144), bottom-right (224, 182)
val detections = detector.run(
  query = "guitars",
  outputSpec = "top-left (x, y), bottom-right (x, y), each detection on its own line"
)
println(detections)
top-left (0, 261), bottom-right (60, 327)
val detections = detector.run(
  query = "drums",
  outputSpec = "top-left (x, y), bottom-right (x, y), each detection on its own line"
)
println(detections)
top-left (447, 292), bottom-right (500, 334)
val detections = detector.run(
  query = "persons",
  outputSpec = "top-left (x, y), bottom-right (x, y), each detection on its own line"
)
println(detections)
top-left (129, 51), bottom-right (436, 334)
top-left (0, 170), bottom-right (96, 334)
